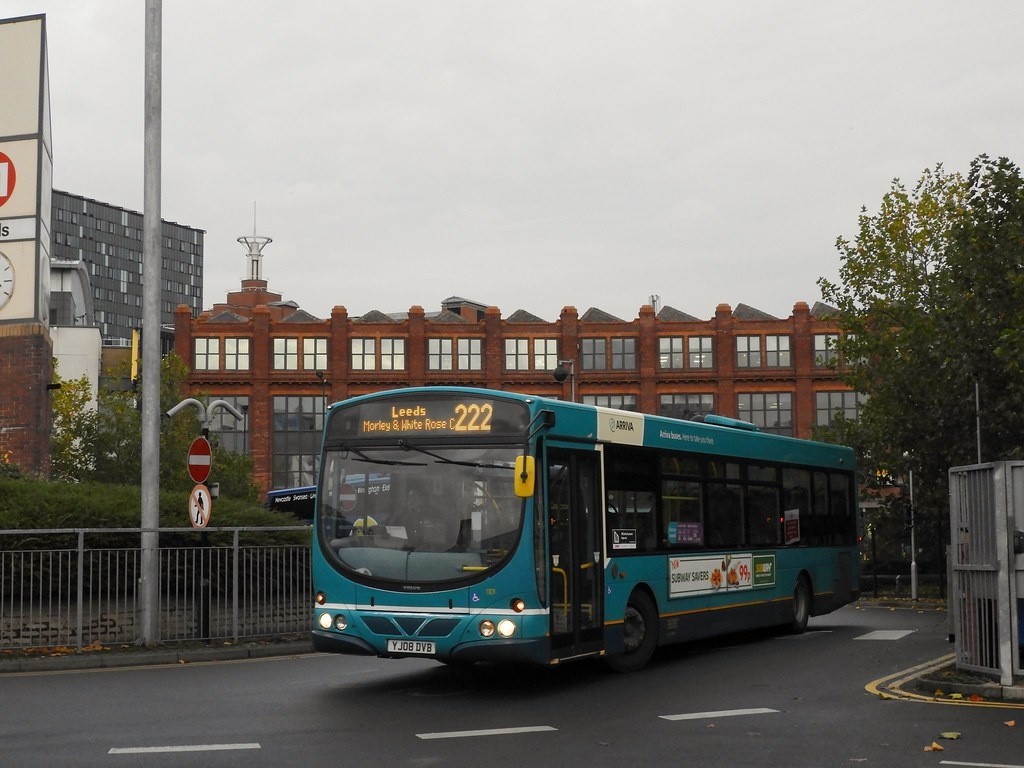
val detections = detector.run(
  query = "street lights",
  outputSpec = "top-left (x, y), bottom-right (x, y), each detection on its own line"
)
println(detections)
top-left (554, 360), bottom-right (574, 402)
top-left (963, 352), bottom-right (981, 464)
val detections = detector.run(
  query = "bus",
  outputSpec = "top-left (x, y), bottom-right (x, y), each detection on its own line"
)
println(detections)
top-left (266, 474), bottom-right (393, 527)
top-left (312, 386), bottom-right (862, 674)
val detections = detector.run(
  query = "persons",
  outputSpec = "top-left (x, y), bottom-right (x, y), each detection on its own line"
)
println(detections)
top-left (382, 485), bottom-right (448, 551)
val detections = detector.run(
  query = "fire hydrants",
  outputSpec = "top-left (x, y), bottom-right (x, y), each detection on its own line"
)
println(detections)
top-left (894, 575), bottom-right (903, 595)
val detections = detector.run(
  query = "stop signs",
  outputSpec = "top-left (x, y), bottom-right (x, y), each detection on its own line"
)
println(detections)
top-left (338, 482), bottom-right (358, 512)
top-left (187, 437), bottom-right (212, 484)
